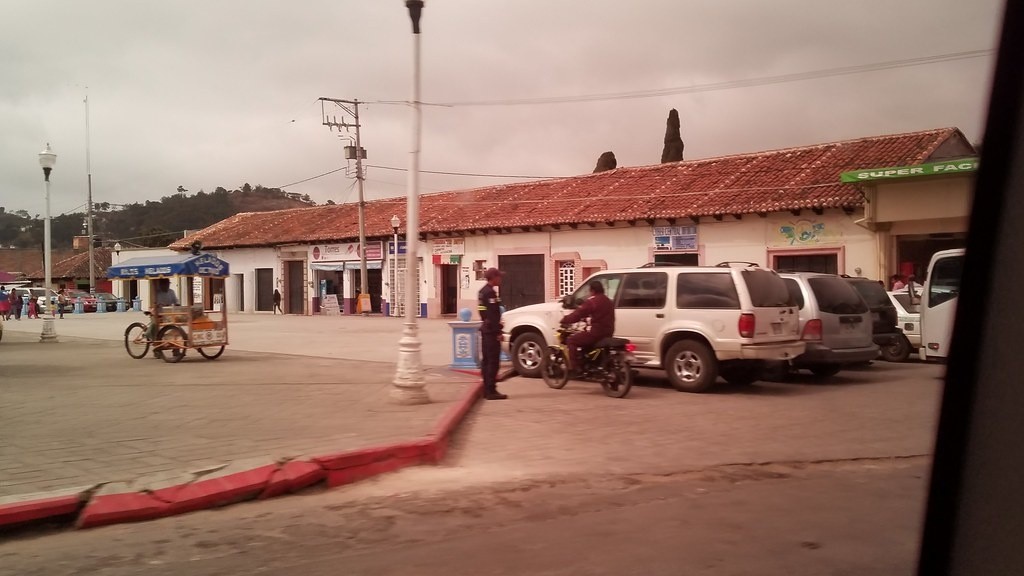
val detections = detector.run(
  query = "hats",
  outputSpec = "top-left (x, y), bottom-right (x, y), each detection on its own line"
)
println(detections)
top-left (159, 278), bottom-right (171, 285)
top-left (485, 267), bottom-right (507, 279)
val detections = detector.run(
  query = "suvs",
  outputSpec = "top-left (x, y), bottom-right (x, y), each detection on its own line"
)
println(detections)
top-left (9, 287), bottom-right (58, 313)
top-left (774, 269), bottom-right (880, 378)
top-left (498, 261), bottom-right (807, 392)
top-left (839, 276), bottom-right (902, 348)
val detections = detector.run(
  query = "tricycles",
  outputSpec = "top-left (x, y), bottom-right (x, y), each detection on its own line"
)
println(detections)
top-left (106, 252), bottom-right (229, 361)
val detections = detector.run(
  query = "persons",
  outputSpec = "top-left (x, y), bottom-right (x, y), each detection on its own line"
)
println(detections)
top-left (891, 275), bottom-right (904, 291)
top-left (561, 281), bottom-right (615, 377)
top-left (58, 290), bottom-right (65, 319)
top-left (273, 289), bottom-right (283, 315)
top-left (51, 301), bottom-right (57, 316)
top-left (155, 278), bottom-right (186, 358)
top-left (478, 267), bottom-right (507, 401)
top-left (0, 285), bottom-right (23, 321)
top-left (27, 296), bottom-right (41, 319)
top-left (905, 275), bottom-right (922, 288)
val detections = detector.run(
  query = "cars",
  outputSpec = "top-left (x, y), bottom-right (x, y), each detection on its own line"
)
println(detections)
top-left (55, 291), bottom-right (91, 313)
top-left (883, 291), bottom-right (921, 362)
top-left (90, 293), bottom-right (129, 312)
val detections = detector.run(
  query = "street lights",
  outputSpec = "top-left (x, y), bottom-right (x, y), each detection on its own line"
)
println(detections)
top-left (38, 143), bottom-right (58, 343)
top-left (391, 214), bottom-right (401, 317)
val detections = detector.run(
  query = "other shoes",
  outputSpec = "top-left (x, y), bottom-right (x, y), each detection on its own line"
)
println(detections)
top-left (566, 367), bottom-right (582, 374)
top-left (58, 309), bottom-right (61, 313)
top-left (282, 312), bottom-right (283, 315)
top-left (60, 317), bottom-right (64, 319)
top-left (483, 389), bottom-right (507, 400)
top-left (172, 350), bottom-right (186, 356)
top-left (154, 351), bottom-right (165, 359)
top-left (16, 317), bottom-right (21, 321)
top-left (3, 318), bottom-right (11, 321)
top-left (28, 316), bottom-right (41, 319)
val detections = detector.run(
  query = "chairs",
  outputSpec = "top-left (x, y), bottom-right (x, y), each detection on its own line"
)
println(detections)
top-left (640, 281), bottom-right (656, 307)
top-left (619, 281), bottom-right (639, 306)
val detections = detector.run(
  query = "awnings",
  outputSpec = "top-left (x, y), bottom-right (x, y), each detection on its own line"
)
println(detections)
top-left (840, 157), bottom-right (981, 202)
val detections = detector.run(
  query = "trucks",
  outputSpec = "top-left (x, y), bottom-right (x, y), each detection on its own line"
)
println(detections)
top-left (919, 249), bottom-right (965, 360)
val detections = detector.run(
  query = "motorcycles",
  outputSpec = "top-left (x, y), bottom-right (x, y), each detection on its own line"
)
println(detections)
top-left (540, 318), bottom-right (636, 399)
